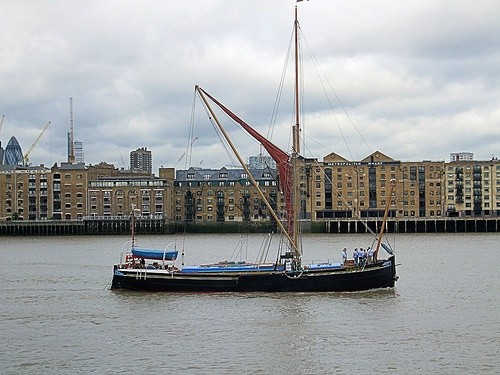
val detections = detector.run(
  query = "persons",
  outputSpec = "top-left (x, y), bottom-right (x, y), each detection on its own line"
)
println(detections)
top-left (342, 247), bottom-right (374, 269)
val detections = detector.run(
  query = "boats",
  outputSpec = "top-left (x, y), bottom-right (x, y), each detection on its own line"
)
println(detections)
top-left (131, 248), bottom-right (179, 261)
top-left (112, 0), bottom-right (397, 293)
